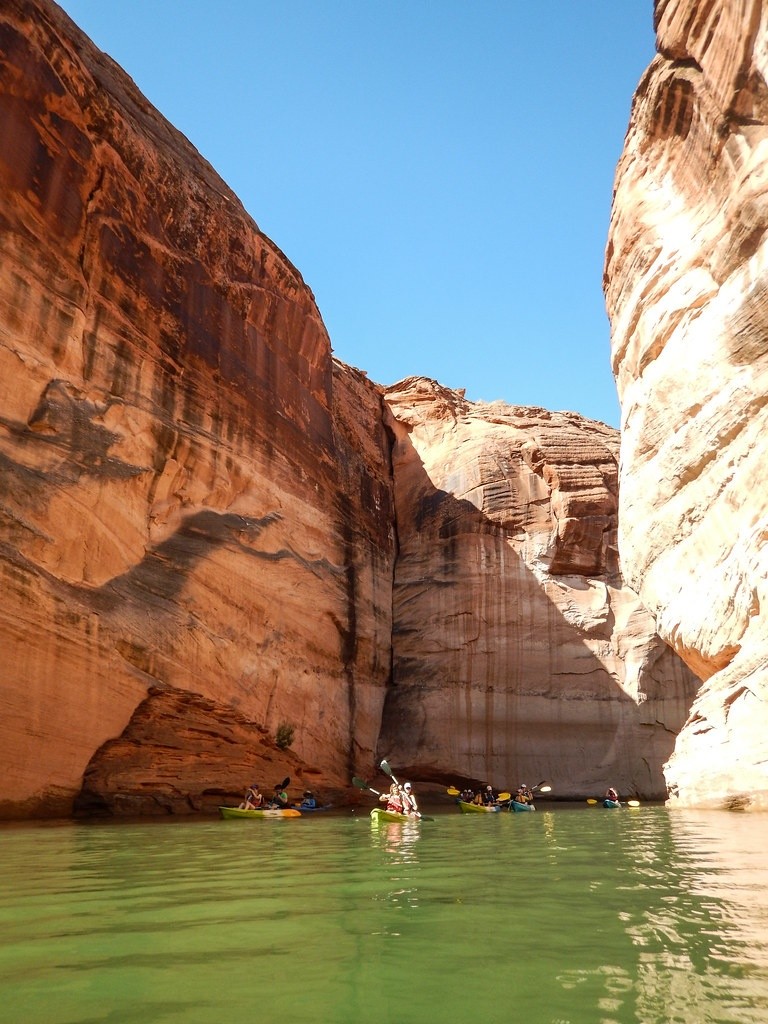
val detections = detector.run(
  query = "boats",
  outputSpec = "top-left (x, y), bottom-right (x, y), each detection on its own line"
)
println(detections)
top-left (497, 793), bottom-right (513, 805)
top-left (291, 802), bottom-right (333, 813)
top-left (509, 798), bottom-right (537, 812)
top-left (371, 807), bottom-right (435, 822)
top-left (218, 804), bottom-right (301, 818)
top-left (457, 800), bottom-right (501, 814)
top-left (602, 799), bottom-right (630, 808)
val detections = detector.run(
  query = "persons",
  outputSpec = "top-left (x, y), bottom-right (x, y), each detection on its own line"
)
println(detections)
top-left (301, 790), bottom-right (316, 809)
top-left (460, 785), bottom-right (495, 807)
top-left (379, 782), bottom-right (418, 814)
top-left (238, 784), bottom-right (263, 810)
top-left (268, 784), bottom-right (288, 810)
top-left (605, 788), bottom-right (618, 802)
top-left (515, 784), bottom-right (533, 805)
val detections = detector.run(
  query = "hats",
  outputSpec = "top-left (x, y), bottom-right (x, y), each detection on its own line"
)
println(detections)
top-left (516, 789), bottom-right (523, 794)
top-left (403, 782), bottom-right (411, 790)
top-left (303, 791), bottom-right (314, 799)
top-left (468, 789), bottom-right (471, 792)
top-left (521, 783), bottom-right (526, 787)
top-left (464, 789), bottom-right (467, 792)
top-left (486, 786), bottom-right (492, 791)
top-left (273, 784), bottom-right (282, 790)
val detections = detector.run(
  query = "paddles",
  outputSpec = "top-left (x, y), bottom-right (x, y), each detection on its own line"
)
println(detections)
top-left (503, 780), bottom-right (544, 806)
top-left (352, 777), bottom-right (434, 821)
top-left (446, 785), bottom-right (511, 802)
top-left (587, 799), bottom-right (640, 806)
top-left (380, 759), bottom-right (420, 815)
top-left (272, 777), bottom-right (291, 804)
